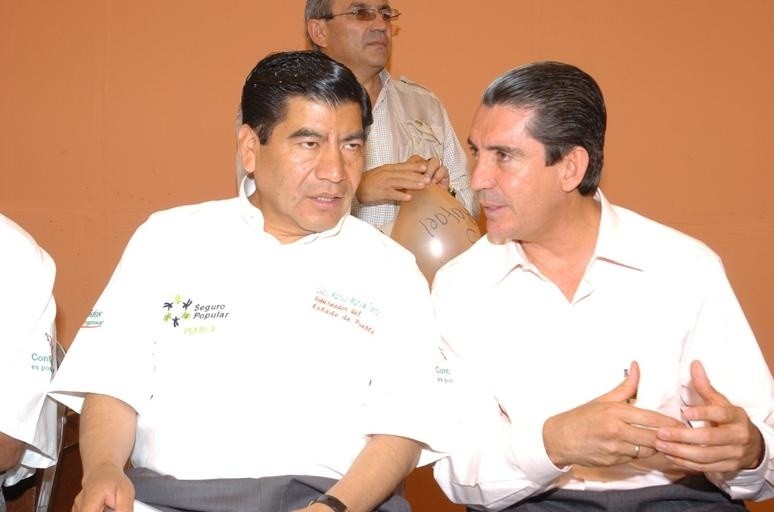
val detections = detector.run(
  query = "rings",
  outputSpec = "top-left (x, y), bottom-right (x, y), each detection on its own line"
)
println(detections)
top-left (633, 444), bottom-right (640, 456)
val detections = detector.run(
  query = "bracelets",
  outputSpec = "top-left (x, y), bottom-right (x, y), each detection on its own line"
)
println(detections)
top-left (308, 495), bottom-right (349, 512)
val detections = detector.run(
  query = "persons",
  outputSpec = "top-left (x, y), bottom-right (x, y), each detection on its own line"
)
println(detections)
top-left (430, 59), bottom-right (773, 511)
top-left (234, 2), bottom-right (475, 240)
top-left (0, 212), bottom-right (60, 510)
top-left (43, 50), bottom-right (445, 512)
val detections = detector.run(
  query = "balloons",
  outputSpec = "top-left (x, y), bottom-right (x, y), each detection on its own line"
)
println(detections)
top-left (391, 155), bottom-right (481, 292)
